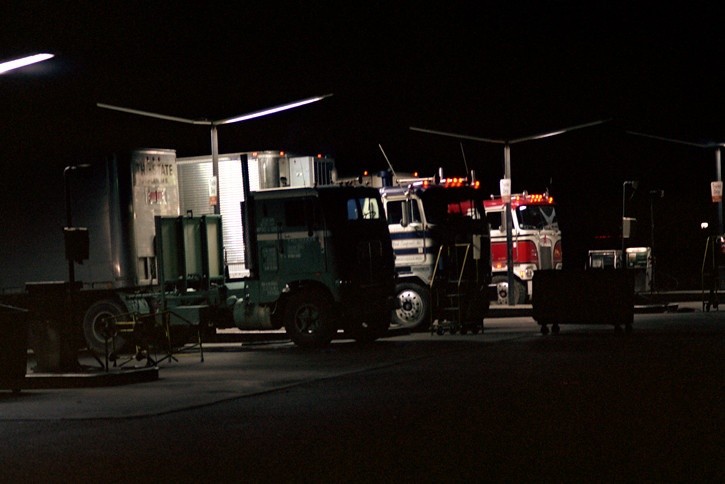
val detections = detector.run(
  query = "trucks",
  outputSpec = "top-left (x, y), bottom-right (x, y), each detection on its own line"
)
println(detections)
top-left (0, 151), bottom-right (498, 351)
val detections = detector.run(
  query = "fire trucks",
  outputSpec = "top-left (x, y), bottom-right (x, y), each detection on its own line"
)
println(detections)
top-left (339, 170), bottom-right (560, 305)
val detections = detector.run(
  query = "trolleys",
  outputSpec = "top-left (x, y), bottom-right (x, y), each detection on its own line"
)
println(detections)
top-left (429, 244), bottom-right (480, 336)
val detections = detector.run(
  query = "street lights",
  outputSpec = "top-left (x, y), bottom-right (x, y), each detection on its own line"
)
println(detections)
top-left (95, 93), bottom-right (334, 275)
top-left (409, 120), bottom-right (606, 308)
